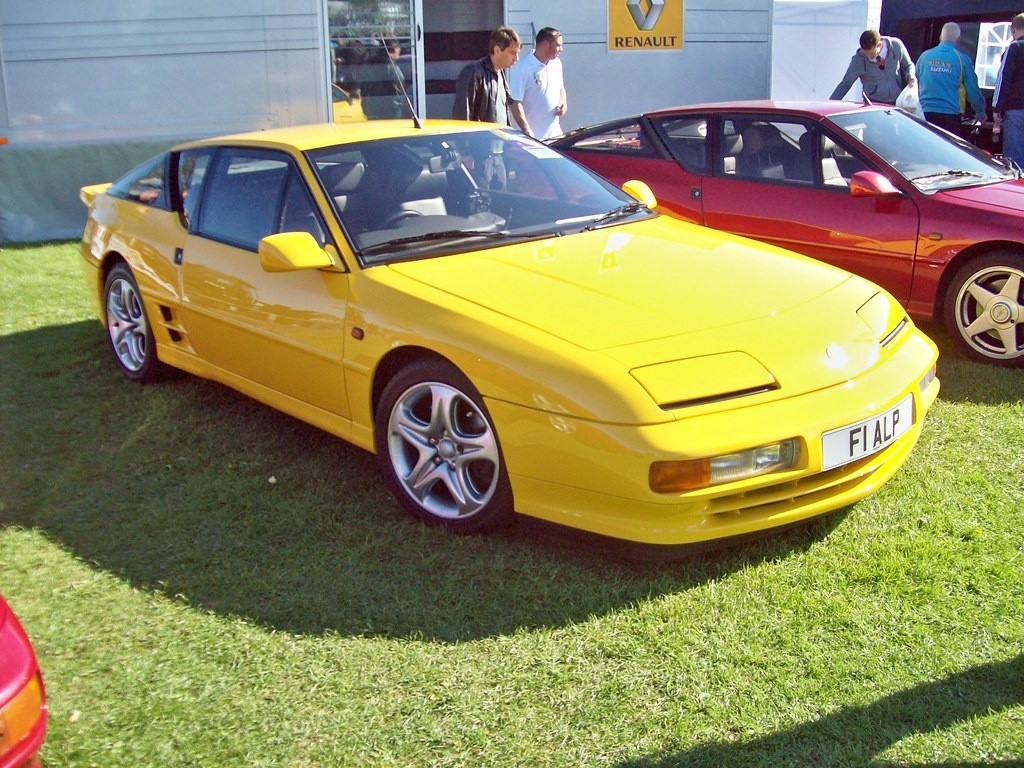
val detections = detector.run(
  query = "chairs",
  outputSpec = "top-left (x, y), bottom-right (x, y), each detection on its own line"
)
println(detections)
top-left (291, 162), bottom-right (383, 246)
top-left (794, 132), bottom-right (835, 181)
top-left (371, 164), bottom-right (448, 215)
top-left (743, 126), bottom-right (785, 179)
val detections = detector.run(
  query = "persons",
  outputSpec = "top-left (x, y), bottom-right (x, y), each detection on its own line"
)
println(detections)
top-left (360, 39), bottom-right (405, 121)
top-left (914, 22), bottom-right (988, 136)
top-left (829, 30), bottom-right (916, 106)
top-left (992, 13), bottom-right (1024, 169)
top-left (451, 27), bottom-right (523, 190)
top-left (333, 32), bottom-right (362, 94)
top-left (508, 28), bottom-right (568, 145)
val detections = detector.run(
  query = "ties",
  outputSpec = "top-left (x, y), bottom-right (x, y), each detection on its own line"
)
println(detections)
top-left (876, 56), bottom-right (885, 70)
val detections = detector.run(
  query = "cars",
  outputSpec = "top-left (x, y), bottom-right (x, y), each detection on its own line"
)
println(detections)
top-left (961, 103), bottom-right (1005, 146)
top-left (539, 97), bottom-right (1024, 368)
top-left (261, 81), bottom-right (369, 132)
top-left (72, 120), bottom-right (941, 546)
top-left (0, 598), bottom-right (47, 768)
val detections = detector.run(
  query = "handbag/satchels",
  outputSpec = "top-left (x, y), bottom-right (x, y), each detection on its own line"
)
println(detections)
top-left (958, 83), bottom-right (966, 113)
top-left (895, 79), bottom-right (926, 121)
